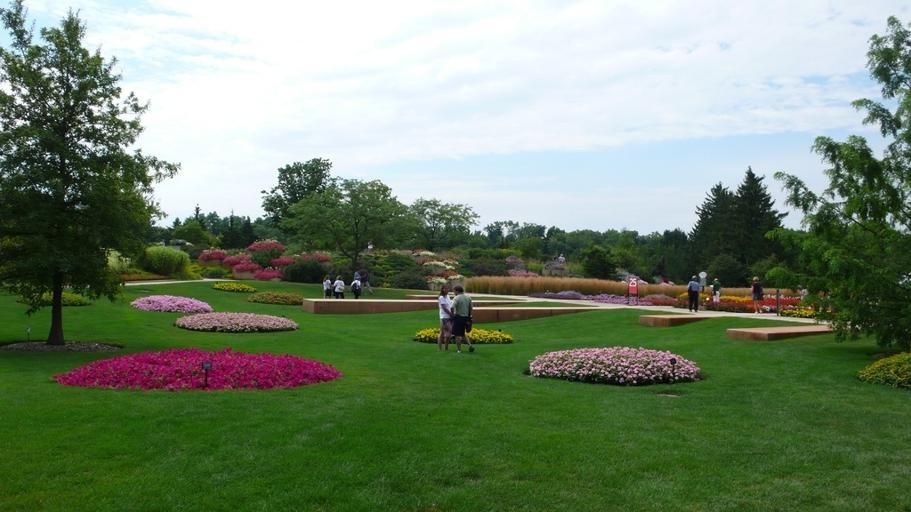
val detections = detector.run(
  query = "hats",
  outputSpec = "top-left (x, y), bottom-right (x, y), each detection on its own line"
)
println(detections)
top-left (692, 275), bottom-right (697, 279)
top-left (752, 276), bottom-right (759, 281)
top-left (714, 278), bottom-right (718, 281)
top-left (354, 271), bottom-right (361, 280)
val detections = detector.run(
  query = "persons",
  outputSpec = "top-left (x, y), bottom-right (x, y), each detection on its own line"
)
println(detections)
top-left (334, 275), bottom-right (346, 299)
top-left (323, 274), bottom-right (333, 299)
top-left (352, 277), bottom-right (362, 299)
top-left (437, 284), bottom-right (455, 352)
top-left (451, 285), bottom-right (476, 353)
top-left (752, 276), bottom-right (765, 313)
top-left (713, 278), bottom-right (722, 302)
top-left (354, 267), bottom-right (374, 295)
top-left (687, 275), bottom-right (700, 312)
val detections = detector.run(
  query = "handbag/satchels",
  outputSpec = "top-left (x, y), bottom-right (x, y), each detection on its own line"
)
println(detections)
top-left (465, 318), bottom-right (473, 333)
top-left (325, 288), bottom-right (332, 296)
top-left (759, 286), bottom-right (764, 297)
top-left (332, 287), bottom-right (336, 296)
top-left (352, 283), bottom-right (362, 293)
top-left (688, 282), bottom-right (692, 296)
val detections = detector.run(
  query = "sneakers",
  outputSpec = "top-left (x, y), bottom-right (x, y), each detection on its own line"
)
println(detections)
top-left (468, 345), bottom-right (475, 353)
top-left (456, 350), bottom-right (462, 354)
